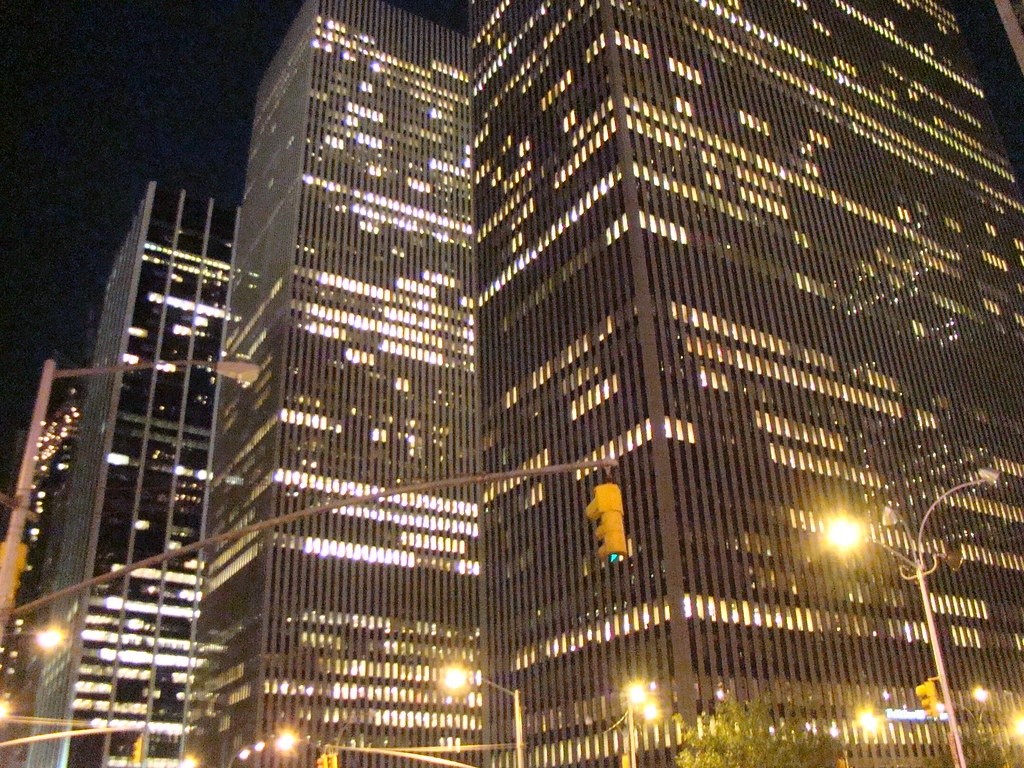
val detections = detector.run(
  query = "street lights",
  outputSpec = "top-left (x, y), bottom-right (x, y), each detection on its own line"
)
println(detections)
top-left (827, 465), bottom-right (1002, 768)
top-left (0, 357), bottom-right (262, 634)
top-left (624, 682), bottom-right (658, 768)
top-left (445, 668), bottom-right (525, 768)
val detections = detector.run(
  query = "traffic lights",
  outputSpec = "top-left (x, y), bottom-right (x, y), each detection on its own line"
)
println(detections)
top-left (915, 678), bottom-right (941, 719)
top-left (834, 749), bottom-right (849, 768)
top-left (585, 483), bottom-right (629, 562)
